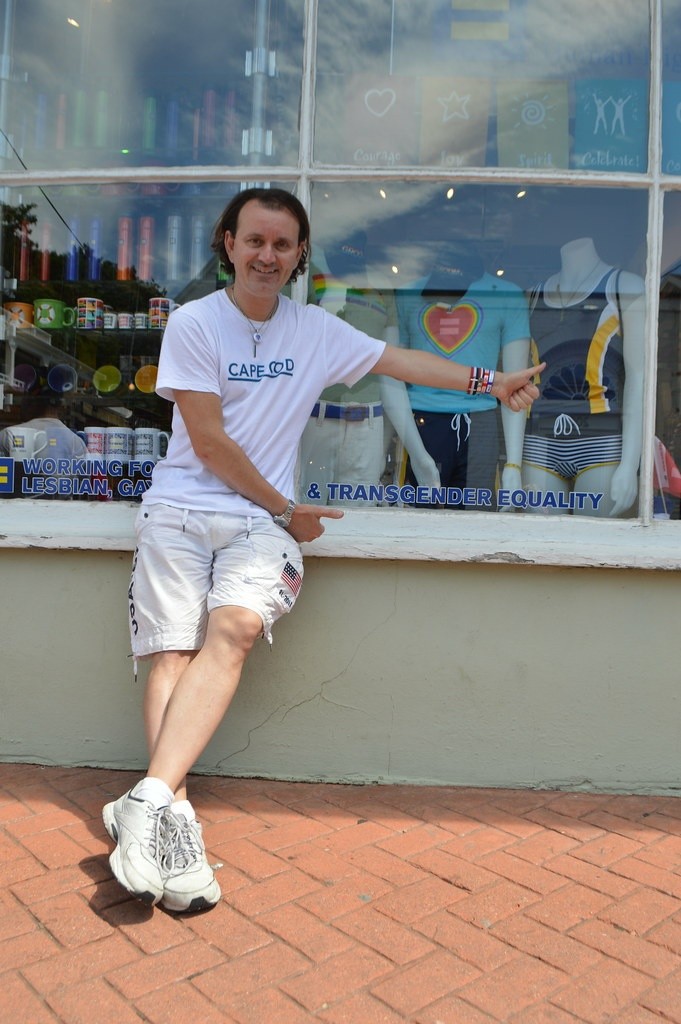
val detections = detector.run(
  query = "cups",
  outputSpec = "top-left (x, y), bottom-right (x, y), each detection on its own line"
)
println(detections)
top-left (148, 298), bottom-right (182, 330)
top-left (3, 302), bottom-right (34, 329)
top-left (70, 297), bottom-right (103, 330)
top-left (104, 305), bottom-right (148, 329)
top-left (34, 299), bottom-right (75, 329)
top-left (75, 427), bottom-right (104, 460)
top-left (104, 427), bottom-right (133, 462)
top-left (46, 427), bottom-right (87, 461)
top-left (7, 427), bottom-right (48, 462)
top-left (134, 427), bottom-right (170, 462)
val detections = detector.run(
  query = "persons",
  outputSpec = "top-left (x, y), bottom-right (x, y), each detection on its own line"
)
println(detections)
top-left (294, 229), bottom-right (441, 507)
top-left (0, 365), bottom-right (91, 484)
top-left (396, 238), bottom-right (531, 512)
top-left (102, 186), bottom-right (544, 911)
top-left (525, 237), bottom-right (644, 516)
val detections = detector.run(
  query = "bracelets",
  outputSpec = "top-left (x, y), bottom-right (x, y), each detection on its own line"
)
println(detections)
top-left (502, 463), bottom-right (521, 473)
top-left (468, 366), bottom-right (494, 396)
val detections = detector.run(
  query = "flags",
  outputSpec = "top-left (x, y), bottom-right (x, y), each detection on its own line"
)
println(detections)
top-left (651, 435), bottom-right (680, 498)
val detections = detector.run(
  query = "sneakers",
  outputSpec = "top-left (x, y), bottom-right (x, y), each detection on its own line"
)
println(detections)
top-left (102, 780), bottom-right (171, 908)
top-left (161, 808), bottom-right (221, 913)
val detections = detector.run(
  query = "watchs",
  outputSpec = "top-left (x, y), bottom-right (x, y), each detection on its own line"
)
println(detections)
top-left (274, 499), bottom-right (295, 529)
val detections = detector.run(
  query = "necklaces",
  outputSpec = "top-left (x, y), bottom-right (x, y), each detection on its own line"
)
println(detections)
top-left (230, 285), bottom-right (279, 358)
top-left (556, 259), bottom-right (603, 320)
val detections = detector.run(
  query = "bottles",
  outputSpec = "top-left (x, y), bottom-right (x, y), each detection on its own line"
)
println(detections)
top-left (136, 216), bottom-right (154, 280)
top-left (65, 217), bottom-right (79, 281)
top-left (88, 216), bottom-right (101, 281)
top-left (39, 220), bottom-right (51, 281)
top-left (26, 81), bottom-right (238, 163)
top-left (166, 215), bottom-right (184, 281)
top-left (18, 220), bottom-right (29, 281)
top-left (187, 215), bottom-right (205, 280)
top-left (115, 217), bottom-right (133, 280)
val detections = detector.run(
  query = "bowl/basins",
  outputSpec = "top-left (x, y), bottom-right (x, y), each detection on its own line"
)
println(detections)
top-left (13, 363), bottom-right (40, 394)
top-left (92, 365), bottom-right (123, 396)
top-left (134, 364), bottom-right (158, 394)
top-left (47, 364), bottom-right (79, 394)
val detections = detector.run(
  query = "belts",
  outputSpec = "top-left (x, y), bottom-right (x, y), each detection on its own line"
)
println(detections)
top-left (310, 403), bottom-right (383, 421)
